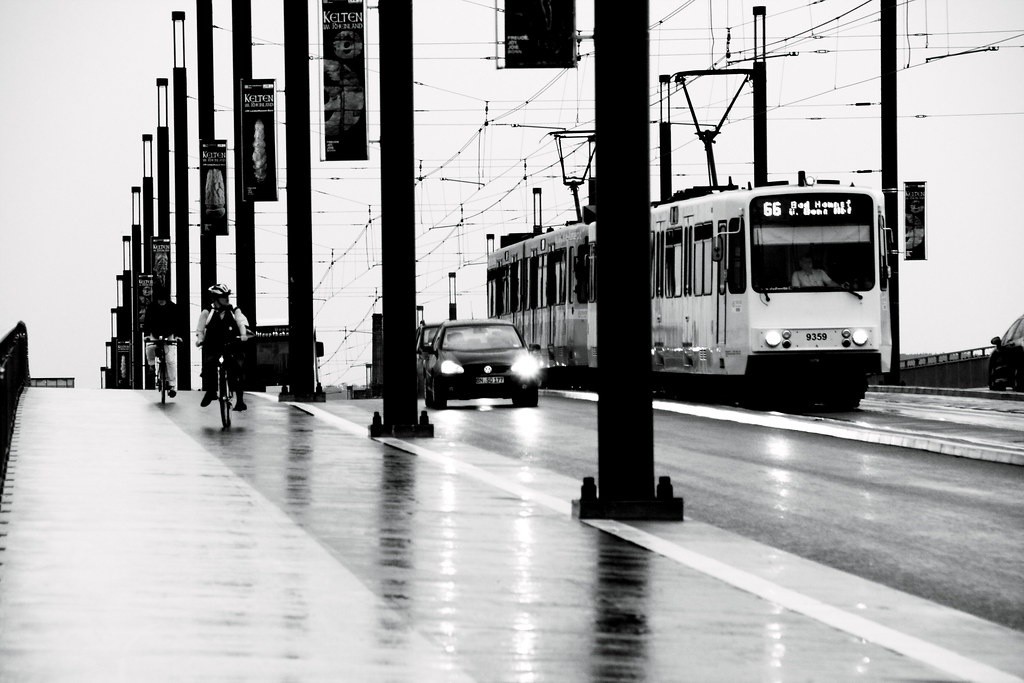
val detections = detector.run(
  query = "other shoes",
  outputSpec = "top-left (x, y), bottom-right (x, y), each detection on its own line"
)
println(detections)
top-left (234, 404), bottom-right (247, 411)
top-left (200, 391), bottom-right (218, 407)
top-left (170, 390), bottom-right (176, 397)
top-left (149, 366), bottom-right (155, 374)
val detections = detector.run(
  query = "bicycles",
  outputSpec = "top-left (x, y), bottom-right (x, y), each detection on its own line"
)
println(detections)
top-left (142, 337), bottom-right (182, 404)
top-left (196, 334), bottom-right (258, 429)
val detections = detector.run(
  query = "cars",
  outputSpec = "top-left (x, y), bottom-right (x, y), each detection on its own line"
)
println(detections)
top-left (415, 317), bottom-right (540, 410)
top-left (987, 316), bottom-right (1023, 395)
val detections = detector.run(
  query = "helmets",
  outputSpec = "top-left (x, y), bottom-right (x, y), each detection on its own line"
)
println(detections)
top-left (208, 283), bottom-right (231, 297)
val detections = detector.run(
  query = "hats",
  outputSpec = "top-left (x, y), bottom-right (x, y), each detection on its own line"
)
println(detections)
top-left (153, 286), bottom-right (170, 300)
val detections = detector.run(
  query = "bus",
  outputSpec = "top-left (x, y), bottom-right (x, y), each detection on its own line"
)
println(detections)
top-left (481, 185), bottom-right (882, 417)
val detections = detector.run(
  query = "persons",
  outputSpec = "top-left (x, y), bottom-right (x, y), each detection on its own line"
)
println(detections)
top-left (142, 286), bottom-right (183, 398)
top-left (195, 285), bottom-right (250, 412)
top-left (791, 254), bottom-right (842, 287)
